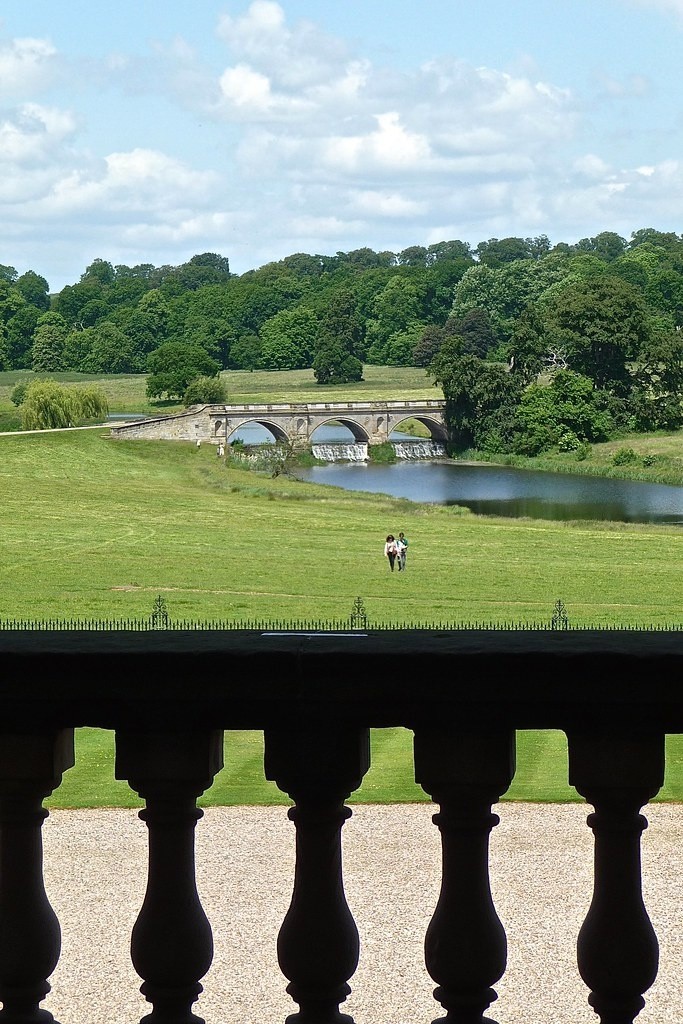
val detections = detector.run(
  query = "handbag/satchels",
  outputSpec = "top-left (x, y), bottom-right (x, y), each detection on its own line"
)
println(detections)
top-left (392, 546), bottom-right (397, 556)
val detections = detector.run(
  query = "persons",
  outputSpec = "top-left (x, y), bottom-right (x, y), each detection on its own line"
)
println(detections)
top-left (397, 532), bottom-right (409, 571)
top-left (196, 440), bottom-right (201, 449)
top-left (217, 443), bottom-right (225, 456)
top-left (384, 535), bottom-right (397, 572)
